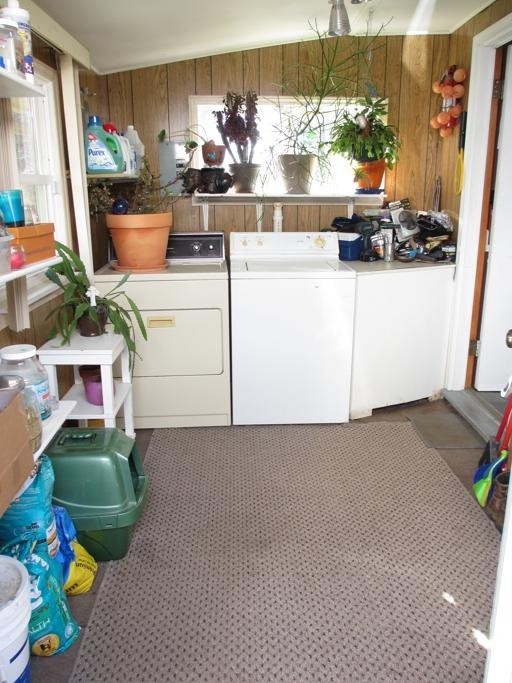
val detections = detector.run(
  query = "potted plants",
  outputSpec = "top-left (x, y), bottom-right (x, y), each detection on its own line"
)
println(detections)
top-left (317, 96), bottom-right (399, 193)
top-left (87, 158), bottom-right (184, 273)
top-left (158, 126), bottom-right (226, 166)
top-left (44, 239), bottom-right (148, 372)
top-left (262, 6), bottom-right (393, 193)
top-left (212, 91), bottom-right (262, 192)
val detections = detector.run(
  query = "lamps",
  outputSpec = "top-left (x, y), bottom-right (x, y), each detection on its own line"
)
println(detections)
top-left (329, 0), bottom-right (351, 36)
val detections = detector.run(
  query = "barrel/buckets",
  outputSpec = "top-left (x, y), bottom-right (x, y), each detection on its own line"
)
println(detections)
top-left (0, 554), bottom-right (32, 683)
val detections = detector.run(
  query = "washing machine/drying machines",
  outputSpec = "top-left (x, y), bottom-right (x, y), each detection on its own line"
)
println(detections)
top-left (85, 114), bottom-right (146, 174)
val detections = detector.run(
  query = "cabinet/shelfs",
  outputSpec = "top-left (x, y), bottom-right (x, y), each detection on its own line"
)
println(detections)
top-left (36, 324), bottom-right (136, 439)
top-left (0, 66), bottom-right (76, 517)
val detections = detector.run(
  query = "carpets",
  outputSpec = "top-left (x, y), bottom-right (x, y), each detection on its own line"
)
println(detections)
top-left (68, 421), bottom-right (501, 683)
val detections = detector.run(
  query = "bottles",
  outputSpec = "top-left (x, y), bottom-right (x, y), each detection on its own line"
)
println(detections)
top-left (0, 344), bottom-right (52, 421)
top-left (0, 375), bottom-right (43, 453)
top-left (0, 0), bottom-right (35, 86)
top-left (369, 233), bottom-right (386, 259)
top-left (0, 18), bottom-right (27, 79)
top-left (383, 243), bottom-right (395, 263)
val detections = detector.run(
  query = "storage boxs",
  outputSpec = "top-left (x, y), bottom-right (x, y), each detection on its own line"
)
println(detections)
top-left (7, 224), bottom-right (55, 265)
top-left (0, 392), bottom-right (35, 519)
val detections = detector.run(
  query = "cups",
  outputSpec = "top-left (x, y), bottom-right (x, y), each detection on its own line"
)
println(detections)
top-left (9, 243), bottom-right (26, 270)
top-left (85, 375), bottom-right (117, 406)
top-left (0, 189), bottom-right (25, 228)
